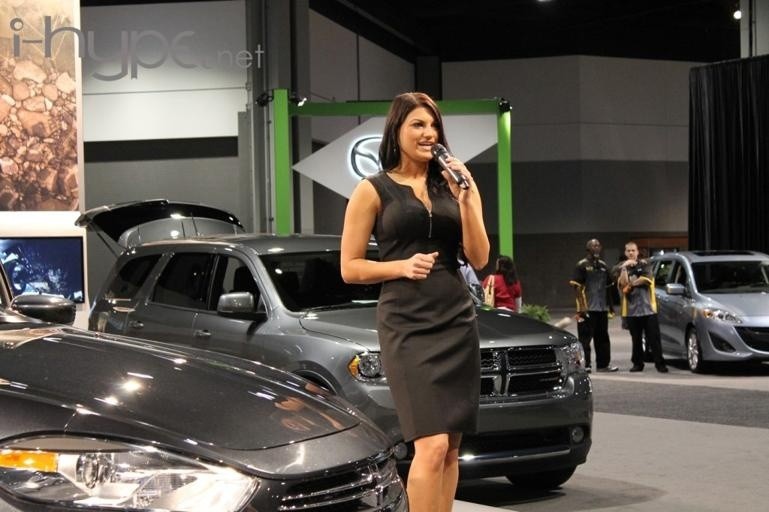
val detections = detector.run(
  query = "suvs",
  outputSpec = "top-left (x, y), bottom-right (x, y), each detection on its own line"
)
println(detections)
top-left (75, 197), bottom-right (594, 494)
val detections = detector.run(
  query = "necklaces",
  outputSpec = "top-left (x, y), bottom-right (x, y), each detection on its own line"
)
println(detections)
top-left (407, 181), bottom-right (428, 199)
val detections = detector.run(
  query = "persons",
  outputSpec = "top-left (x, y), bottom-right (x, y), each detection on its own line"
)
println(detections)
top-left (481, 255), bottom-right (522, 317)
top-left (459, 263), bottom-right (484, 308)
top-left (341, 92), bottom-right (491, 512)
top-left (617, 243), bottom-right (671, 373)
top-left (570, 240), bottom-right (618, 374)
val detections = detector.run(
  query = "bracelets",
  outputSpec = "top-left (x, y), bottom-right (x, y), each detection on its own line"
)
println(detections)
top-left (629, 283), bottom-right (634, 288)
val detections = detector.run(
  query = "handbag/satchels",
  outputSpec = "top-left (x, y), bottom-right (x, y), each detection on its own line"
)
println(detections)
top-left (483, 274), bottom-right (496, 309)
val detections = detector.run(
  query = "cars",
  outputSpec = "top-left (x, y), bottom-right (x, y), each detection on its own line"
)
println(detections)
top-left (0, 260), bottom-right (410, 510)
top-left (646, 251), bottom-right (768, 373)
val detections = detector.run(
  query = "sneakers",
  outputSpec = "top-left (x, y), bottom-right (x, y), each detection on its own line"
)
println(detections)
top-left (595, 365), bottom-right (618, 372)
top-left (585, 363), bottom-right (592, 372)
top-left (629, 363), bottom-right (645, 372)
top-left (656, 363), bottom-right (668, 373)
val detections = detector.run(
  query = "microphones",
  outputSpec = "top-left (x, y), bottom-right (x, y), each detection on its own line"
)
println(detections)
top-left (432, 144), bottom-right (467, 188)
